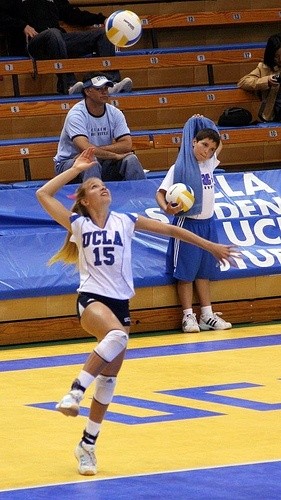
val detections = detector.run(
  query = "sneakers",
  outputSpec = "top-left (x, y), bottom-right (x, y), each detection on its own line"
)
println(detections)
top-left (74, 440), bottom-right (97, 475)
top-left (55, 390), bottom-right (84, 417)
top-left (198, 311), bottom-right (232, 331)
top-left (182, 313), bottom-right (200, 333)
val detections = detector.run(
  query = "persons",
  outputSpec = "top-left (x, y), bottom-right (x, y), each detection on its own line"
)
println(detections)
top-left (237, 34), bottom-right (281, 123)
top-left (35, 147), bottom-right (242, 475)
top-left (156, 115), bottom-right (232, 333)
top-left (0, 0), bottom-right (133, 96)
top-left (52, 72), bottom-right (146, 183)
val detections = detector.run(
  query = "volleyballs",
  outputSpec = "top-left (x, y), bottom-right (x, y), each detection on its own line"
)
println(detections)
top-left (105, 8), bottom-right (142, 49)
top-left (165, 182), bottom-right (196, 214)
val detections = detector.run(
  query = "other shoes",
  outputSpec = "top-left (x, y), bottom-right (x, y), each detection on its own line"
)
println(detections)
top-left (68, 81), bottom-right (84, 96)
top-left (108, 77), bottom-right (133, 95)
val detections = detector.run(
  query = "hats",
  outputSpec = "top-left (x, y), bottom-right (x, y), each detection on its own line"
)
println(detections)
top-left (82, 76), bottom-right (114, 89)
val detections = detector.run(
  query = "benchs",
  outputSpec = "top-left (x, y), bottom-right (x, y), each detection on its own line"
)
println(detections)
top-left (0, 0), bottom-right (281, 188)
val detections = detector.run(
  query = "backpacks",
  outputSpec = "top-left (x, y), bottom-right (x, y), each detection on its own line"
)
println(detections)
top-left (218, 107), bottom-right (252, 126)
